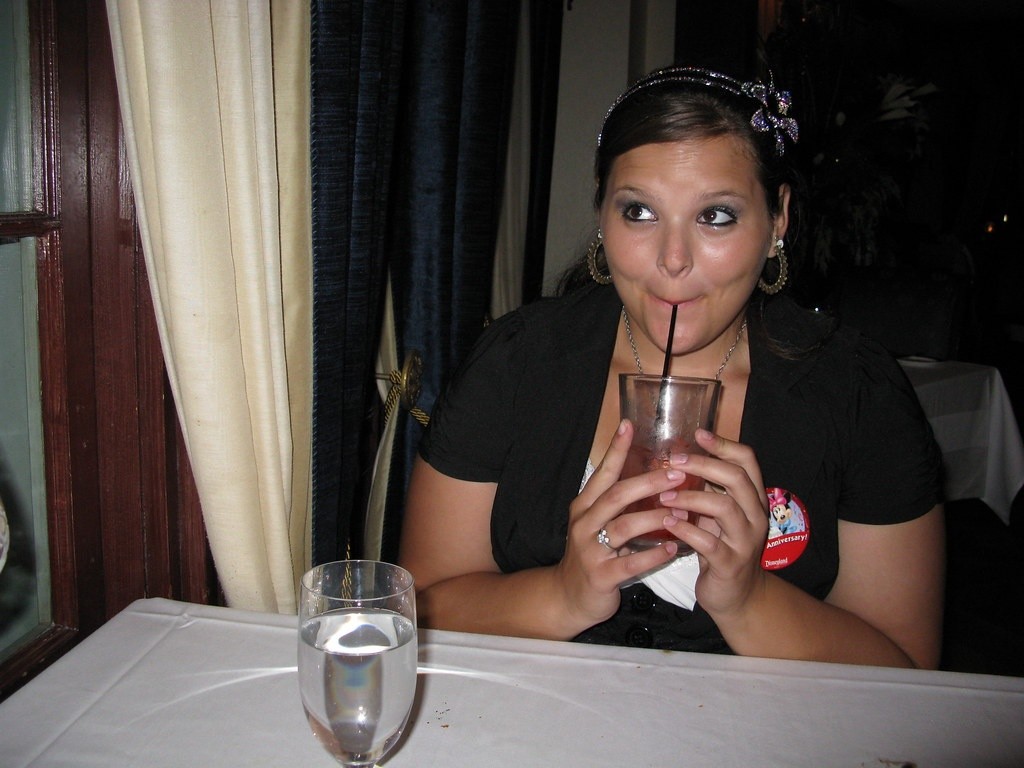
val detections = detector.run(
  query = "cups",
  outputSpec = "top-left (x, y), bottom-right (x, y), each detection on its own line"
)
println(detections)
top-left (299, 561), bottom-right (419, 768)
top-left (619, 372), bottom-right (724, 550)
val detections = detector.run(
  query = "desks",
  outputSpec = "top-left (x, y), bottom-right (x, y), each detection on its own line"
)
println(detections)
top-left (0, 597), bottom-right (1024, 768)
top-left (895, 355), bottom-right (1024, 529)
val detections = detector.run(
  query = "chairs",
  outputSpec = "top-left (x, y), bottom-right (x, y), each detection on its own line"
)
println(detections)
top-left (838, 286), bottom-right (952, 367)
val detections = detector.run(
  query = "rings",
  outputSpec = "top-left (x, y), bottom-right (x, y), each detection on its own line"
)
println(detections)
top-left (597, 529), bottom-right (614, 551)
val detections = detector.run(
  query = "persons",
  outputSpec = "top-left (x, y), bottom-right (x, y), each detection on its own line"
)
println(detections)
top-left (392, 61), bottom-right (946, 678)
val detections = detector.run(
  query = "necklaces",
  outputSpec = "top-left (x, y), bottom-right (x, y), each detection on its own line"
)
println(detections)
top-left (623, 307), bottom-right (747, 384)
